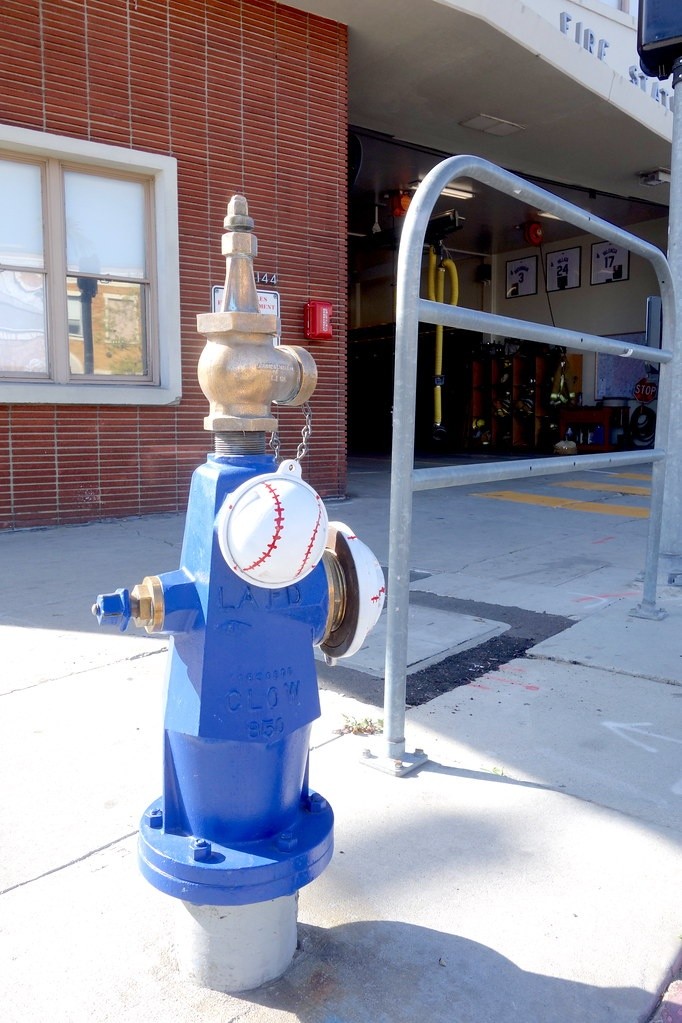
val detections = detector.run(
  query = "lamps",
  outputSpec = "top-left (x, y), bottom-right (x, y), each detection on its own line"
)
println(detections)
top-left (409, 169), bottom-right (477, 201)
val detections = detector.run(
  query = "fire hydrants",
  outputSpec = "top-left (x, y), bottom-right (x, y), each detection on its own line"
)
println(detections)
top-left (93, 194), bottom-right (384, 913)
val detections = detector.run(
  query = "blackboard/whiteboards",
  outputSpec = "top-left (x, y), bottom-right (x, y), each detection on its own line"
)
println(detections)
top-left (593, 331), bottom-right (660, 399)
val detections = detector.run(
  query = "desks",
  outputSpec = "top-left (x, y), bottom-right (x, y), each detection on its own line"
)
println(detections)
top-left (559, 405), bottom-right (630, 451)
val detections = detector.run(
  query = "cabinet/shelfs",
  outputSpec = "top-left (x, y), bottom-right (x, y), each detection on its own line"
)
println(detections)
top-left (468, 349), bottom-right (546, 456)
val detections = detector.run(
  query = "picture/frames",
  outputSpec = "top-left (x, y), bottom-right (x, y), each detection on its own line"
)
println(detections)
top-left (505, 254), bottom-right (538, 299)
top-left (590, 240), bottom-right (630, 286)
top-left (545, 245), bottom-right (581, 293)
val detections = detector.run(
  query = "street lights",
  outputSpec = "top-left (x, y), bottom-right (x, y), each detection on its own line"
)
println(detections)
top-left (627, 0), bottom-right (682, 552)
